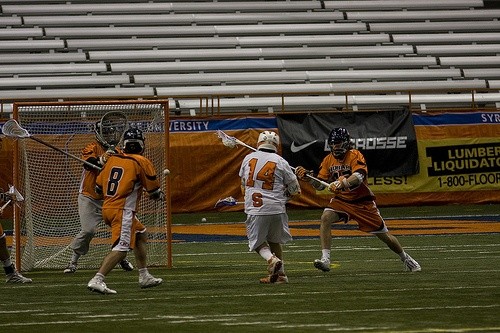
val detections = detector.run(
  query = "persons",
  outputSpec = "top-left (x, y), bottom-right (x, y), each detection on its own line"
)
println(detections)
top-left (0, 188), bottom-right (33, 284)
top-left (63, 120), bottom-right (133, 274)
top-left (88, 128), bottom-right (165, 294)
top-left (295, 127), bottom-right (422, 272)
top-left (239, 131), bottom-right (301, 283)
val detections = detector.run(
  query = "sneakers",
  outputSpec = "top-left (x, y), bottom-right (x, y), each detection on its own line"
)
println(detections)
top-left (6, 274), bottom-right (32, 283)
top-left (139, 274), bottom-right (162, 288)
top-left (260, 276), bottom-right (290, 284)
top-left (88, 279), bottom-right (117, 294)
top-left (314, 260), bottom-right (331, 271)
top-left (118, 258), bottom-right (135, 271)
top-left (63, 264), bottom-right (76, 273)
top-left (267, 255), bottom-right (283, 283)
top-left (401, 253), bottom-right (421, 271)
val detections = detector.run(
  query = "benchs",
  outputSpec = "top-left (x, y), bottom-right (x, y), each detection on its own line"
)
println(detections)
top-left (0, 0), bottom-right (500, 112)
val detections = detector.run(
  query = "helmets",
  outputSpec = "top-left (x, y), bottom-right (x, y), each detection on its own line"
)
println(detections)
top-left (257, 131), bottom-right (279, 150)
top-left (94, 120), bottom-right (117, 146)
top-left (329, 128), bottom-right (350, 159)
top-left (122, 128), bottom-right (144, 154)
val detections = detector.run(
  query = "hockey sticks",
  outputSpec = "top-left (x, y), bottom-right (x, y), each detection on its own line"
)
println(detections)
top-left (1, 119), bottom-right (148, 193)
top-left (93, 110), bottom-right (130, 152)
top-left (214, 195), bottom-right (245, 208)
top-left (213, 129), bottom-right (330, 188)
top-left (2, 183), bottom-right (24, 210)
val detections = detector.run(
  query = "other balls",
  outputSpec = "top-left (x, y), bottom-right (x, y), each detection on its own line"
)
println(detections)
top-left (202, 218), bottom-right (206, 222)
top-left (164, 169), bottom-right (170, 175)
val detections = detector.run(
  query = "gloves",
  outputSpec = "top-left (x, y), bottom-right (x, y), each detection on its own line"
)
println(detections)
top-left (329, 176), bottom-right (350, 193)
top-left (99, 150), bottom-right (115, 165)
top-left (296, 166), bottom-right (314, 183)
top-left (149, 188), bottom-right (166, 201)
